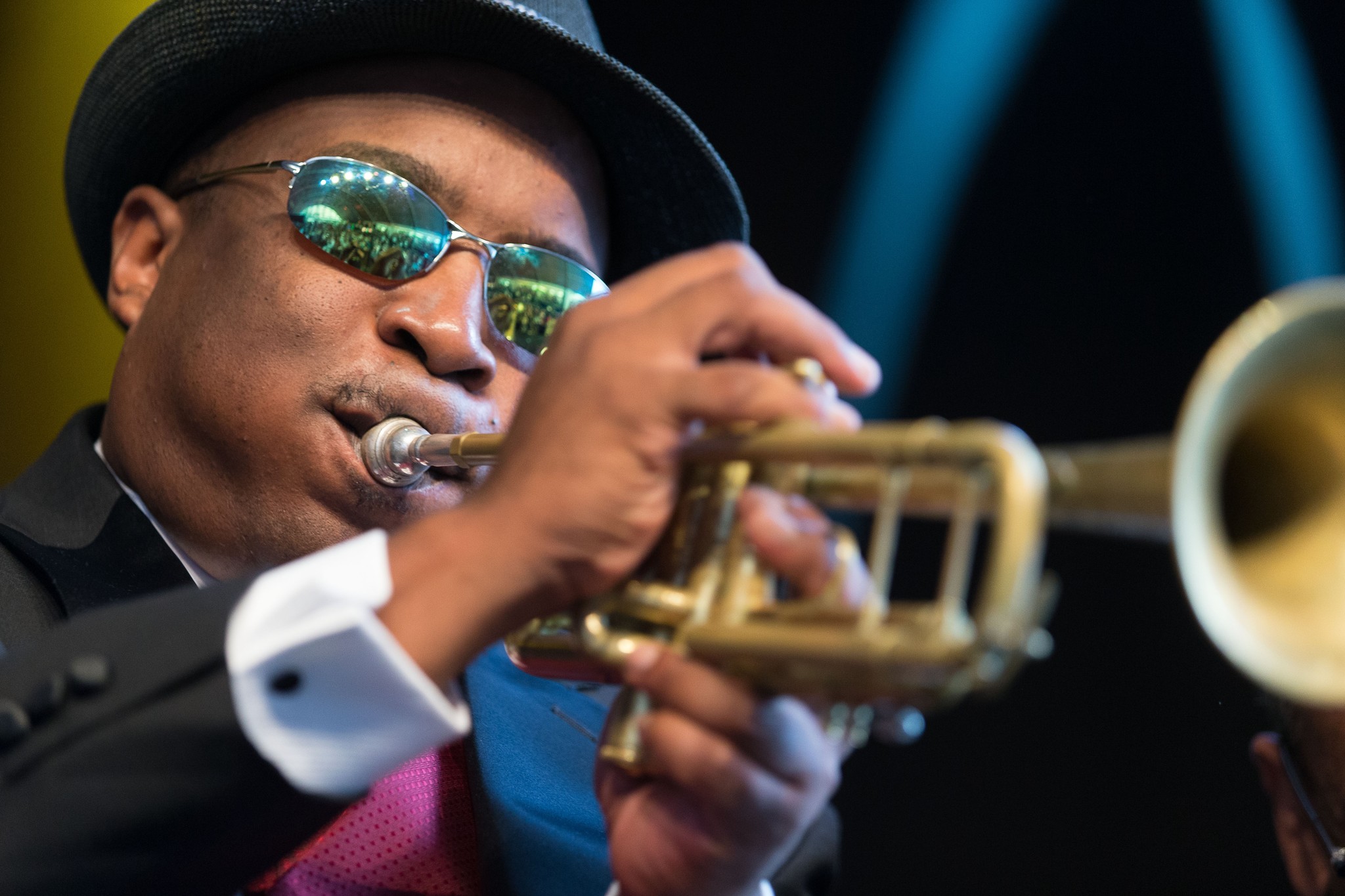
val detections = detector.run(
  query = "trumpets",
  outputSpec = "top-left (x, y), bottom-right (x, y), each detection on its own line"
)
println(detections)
top-left (358, 271), bottom-right (1342, 796)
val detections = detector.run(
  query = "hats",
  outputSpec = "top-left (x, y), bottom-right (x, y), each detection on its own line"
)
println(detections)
top-left (64, 0), bottom-right (749, 337)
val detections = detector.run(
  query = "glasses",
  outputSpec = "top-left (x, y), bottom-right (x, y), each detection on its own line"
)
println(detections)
top-left (165, 153), bottom-right (612, 359)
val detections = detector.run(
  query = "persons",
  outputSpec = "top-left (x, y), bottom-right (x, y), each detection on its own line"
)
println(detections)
top-left (0, 0), bottom-right (881, 895)
